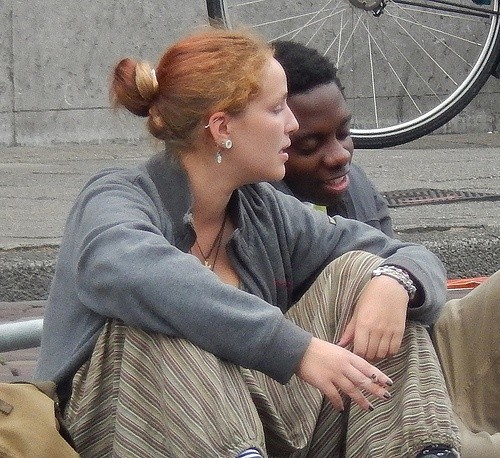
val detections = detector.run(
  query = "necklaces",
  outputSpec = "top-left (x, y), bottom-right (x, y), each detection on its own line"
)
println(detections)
top-left (189, 213), bottom-right (228, 272)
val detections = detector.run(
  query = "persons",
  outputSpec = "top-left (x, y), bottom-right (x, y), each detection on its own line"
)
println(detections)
top-left (32, 30), bottom-right (462, 458)
top-left (268, 41), bottom-right (500, 458)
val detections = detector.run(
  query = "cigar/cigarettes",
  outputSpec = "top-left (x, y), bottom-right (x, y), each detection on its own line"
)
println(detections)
top-left (371, 373), bottom-right (378, 383)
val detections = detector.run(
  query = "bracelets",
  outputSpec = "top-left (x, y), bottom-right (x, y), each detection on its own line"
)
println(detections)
top-left (371, 265), bottom-right (417, 299)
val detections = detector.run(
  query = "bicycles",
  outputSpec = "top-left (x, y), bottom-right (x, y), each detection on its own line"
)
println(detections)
top-left (205, 0), bottom-right (499, 149)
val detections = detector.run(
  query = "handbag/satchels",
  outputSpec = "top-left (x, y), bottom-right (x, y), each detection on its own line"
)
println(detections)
top-left (0, 381), bottom-right (80, 458)
